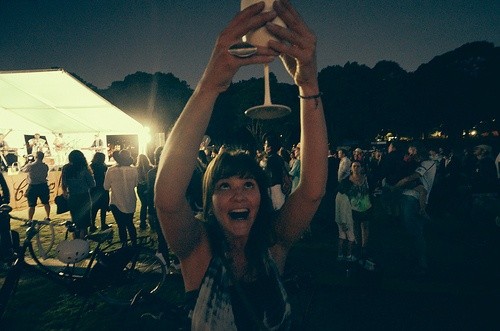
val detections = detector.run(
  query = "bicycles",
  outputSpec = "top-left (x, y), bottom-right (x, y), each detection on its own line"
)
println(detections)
top-left (0, 202), bottom-right (170, 325)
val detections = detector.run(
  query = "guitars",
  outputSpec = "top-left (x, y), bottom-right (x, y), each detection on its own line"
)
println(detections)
top-left (55, 143), bottom-right (73, 151)
top-left (81, 146), bottom-right (103, 151)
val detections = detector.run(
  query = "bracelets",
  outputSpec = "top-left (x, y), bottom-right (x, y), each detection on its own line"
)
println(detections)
top-left (298, 93), bottom-right (321, 109)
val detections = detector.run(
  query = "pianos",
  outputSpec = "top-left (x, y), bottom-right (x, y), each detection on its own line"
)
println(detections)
top-left (0, 148), bottom-right (24, 170)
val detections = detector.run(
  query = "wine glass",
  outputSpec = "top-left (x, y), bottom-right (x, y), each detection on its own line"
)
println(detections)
top-left (241, 0), bottom-right (292, 119)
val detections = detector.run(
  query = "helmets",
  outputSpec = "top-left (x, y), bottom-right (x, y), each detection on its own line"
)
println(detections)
top-left (55, 239), bottom-right (90, 264)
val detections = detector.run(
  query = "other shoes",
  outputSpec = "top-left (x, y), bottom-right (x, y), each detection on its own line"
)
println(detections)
top-left (102, 224), bottom-right (112, 231)
top-left (89, 226), bottom-right (98, 233)
top-left (20, 220), bottom-right (33, 227)
top-left (338, 254), bottom-right (344, 260)
top-left (344, 255), bottom-right (357, 262)
top-left (45, 217), bottom-right (51, 221)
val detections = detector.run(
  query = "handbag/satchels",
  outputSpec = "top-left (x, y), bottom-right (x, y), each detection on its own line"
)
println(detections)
top-left (55, 193), bottom-right (71, 214)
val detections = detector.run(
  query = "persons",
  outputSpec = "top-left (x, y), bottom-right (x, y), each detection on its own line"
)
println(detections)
top-left (62, 151), bottom-right (96, 241)
top-left (91, 135), bottom-right (104, 152)
top-left (55, 133), bottom-right (64, 152)
top-left (103, 150), bottom-right (139, 249)
top-left (25, 152), bottom-right (52, 225)
top-left (136, 125), bottom-right (500, 263)
top-left (0, 134), bottom-right (8, 163)
top-left (90, 152), bottom-right (112, 232)
top-left (153, 0), bottom-right (329, 330)
top-left (31, 134), bottom-right (47, 160)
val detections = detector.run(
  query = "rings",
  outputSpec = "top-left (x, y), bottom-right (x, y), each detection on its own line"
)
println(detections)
top-left (227, 42), bottom-right (259, 58)
top-left (292, 37), bottom-right (303, 49)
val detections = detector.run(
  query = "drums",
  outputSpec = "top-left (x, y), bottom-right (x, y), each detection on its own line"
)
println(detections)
top-left (28, 154), bottom-right (35, 163)
top-left (44, 157), bottom-right (56, 166)
top-left (37, 146), bottom-right (44, 152)
